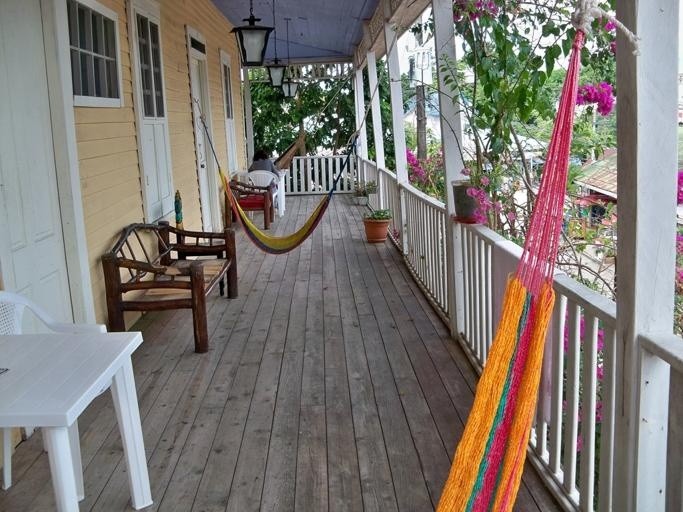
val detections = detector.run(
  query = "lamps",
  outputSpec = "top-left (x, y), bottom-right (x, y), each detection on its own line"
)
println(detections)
top-left (229, 0), bottom-right (300, 99)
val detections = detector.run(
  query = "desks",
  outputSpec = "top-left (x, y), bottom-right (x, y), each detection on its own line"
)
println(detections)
top-left (240, 170), bottom-right (285, 216)
top-left (0, 332), bottom-right (154, 512)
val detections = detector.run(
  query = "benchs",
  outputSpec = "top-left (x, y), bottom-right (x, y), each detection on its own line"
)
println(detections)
top-left (101, 222), bottom-right (238, 354)
top-left (224, 180), bottom-right (274, 230)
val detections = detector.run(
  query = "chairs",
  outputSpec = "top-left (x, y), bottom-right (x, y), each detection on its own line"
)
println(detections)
top-left (248, 170), bottom-right (282, 221)
top-left (0, 291), bottom-right (108, 502)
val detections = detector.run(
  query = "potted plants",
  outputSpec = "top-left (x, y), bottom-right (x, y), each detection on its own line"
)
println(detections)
top-left (354, 179), bottom-right (376, 205)
top-left (363, 209), bottom-right (393, 244)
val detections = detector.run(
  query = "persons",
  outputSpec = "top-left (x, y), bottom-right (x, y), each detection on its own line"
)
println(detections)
top-left (248, 150), bottom-right (280, 213)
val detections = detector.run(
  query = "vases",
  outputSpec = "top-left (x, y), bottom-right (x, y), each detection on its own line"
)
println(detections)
top-left (451, 179), bottom-right (480, 223)
